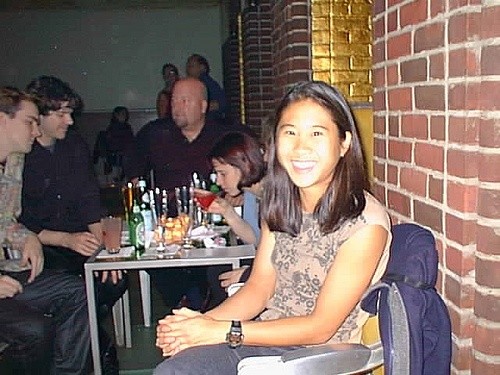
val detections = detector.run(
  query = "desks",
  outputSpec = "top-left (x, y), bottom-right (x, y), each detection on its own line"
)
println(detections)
top-left (83, 226), bottom-right (256, 375)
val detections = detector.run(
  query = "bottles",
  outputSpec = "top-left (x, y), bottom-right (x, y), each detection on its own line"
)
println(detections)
top-left (174, 171), bottom-right (221, 223)
top-left (128, 199), bottom-right (145, 256)
top-left (123, 179), bottom-right (167, 232)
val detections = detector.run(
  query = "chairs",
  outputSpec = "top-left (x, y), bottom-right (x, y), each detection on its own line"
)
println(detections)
top-left (227, 223), bottom-right (439, 375)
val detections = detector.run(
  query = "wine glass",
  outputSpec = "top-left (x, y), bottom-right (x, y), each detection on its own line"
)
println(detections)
top-left (194, 188), bottom-right (217, 227)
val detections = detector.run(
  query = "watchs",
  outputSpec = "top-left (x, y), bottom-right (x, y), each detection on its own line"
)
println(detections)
top-left (226, 321), bottom-right (244, 348)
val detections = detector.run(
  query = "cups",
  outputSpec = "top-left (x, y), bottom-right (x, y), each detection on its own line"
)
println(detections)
top-left (100, 218), bottom-right (122, 253)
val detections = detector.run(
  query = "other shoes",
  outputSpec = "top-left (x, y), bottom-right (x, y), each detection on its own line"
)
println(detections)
top-left (102, 345), bottom-right (119, 375)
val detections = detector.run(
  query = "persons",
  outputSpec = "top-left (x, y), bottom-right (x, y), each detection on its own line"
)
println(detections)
top-left (90, 77), bottom-right (255, 317)
top-left (105, 53), bottom-right (227, 196)
top-left (16, 76), bottom-right (129, 375)
top-left (194, 113), bottom-right (275, 293)
top-left (0, 86), bottom-right (92, 375)
top-left (152, 81), bottom-right (393, 375)
